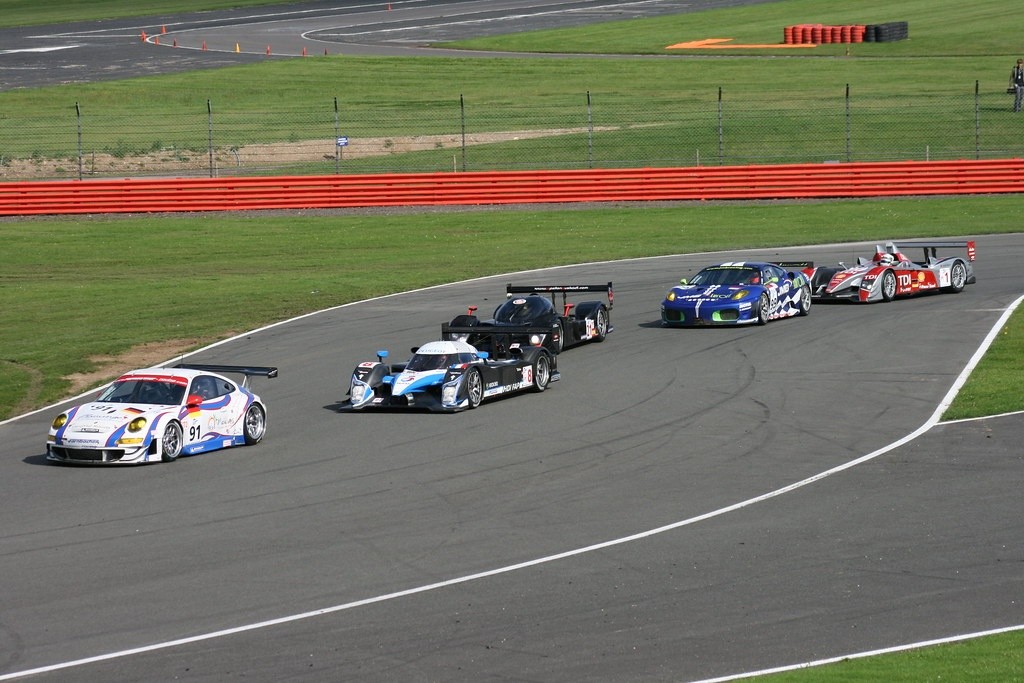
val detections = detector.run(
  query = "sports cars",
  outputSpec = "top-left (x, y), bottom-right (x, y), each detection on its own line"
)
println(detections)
top-left (661, 260), bottom-right (814, 327)
top-left (43, 363), bottom-right (276, 466)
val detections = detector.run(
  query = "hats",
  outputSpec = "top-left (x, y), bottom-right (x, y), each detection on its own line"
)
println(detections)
top-left (1016, 58), bottom-right (1023, 64)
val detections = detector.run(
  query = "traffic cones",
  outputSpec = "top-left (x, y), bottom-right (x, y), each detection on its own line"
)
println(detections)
top-left (266, 45), bottom-right (272, 56)
top-left (235, 43), bottom-right (240, 53)
top-left (302, 47), bottom-right (307, 57)
top-left (173, 38), bottom-right (178, 48)
top-left (161, 25), bottom-right (167, 35)
top-left (155, 36), bottom-right (159, 45)
top-left (141, 30), bottom-right (146, 42)
top-left (324, 48), bottom-right (328, 55)
top-left (388, 3), bottom-right (392, 11)
top-left (202, 41), bottom-right (208, 50)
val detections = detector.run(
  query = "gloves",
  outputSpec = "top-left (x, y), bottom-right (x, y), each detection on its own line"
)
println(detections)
top-left (1015, 84), bottom-right (1018, 88)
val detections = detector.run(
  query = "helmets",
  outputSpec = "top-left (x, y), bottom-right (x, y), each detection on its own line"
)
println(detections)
top-left (879, 253), bottom-right (894, 266)
top-left (745, 271), bottom-right (760, 284)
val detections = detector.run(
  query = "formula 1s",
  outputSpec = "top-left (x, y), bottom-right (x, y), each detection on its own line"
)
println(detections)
top-left (338, 322), bottom-right (559, 412)
top-left (440, 282), bottom-right (613, 360)
top-left (800, 240), bottom-right (979, 305)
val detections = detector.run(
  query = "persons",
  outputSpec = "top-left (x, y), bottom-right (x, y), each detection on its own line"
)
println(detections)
top-left (1007, 59), bottom-right (1024, 112)
top-left (880, 254), bottom-right (894, 266)
top-left (752, 272), bottom-right (759, 283)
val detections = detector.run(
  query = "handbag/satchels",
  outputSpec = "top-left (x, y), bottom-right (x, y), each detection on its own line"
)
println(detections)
top-left (1006, 87), bottom-right (1017, 95)
